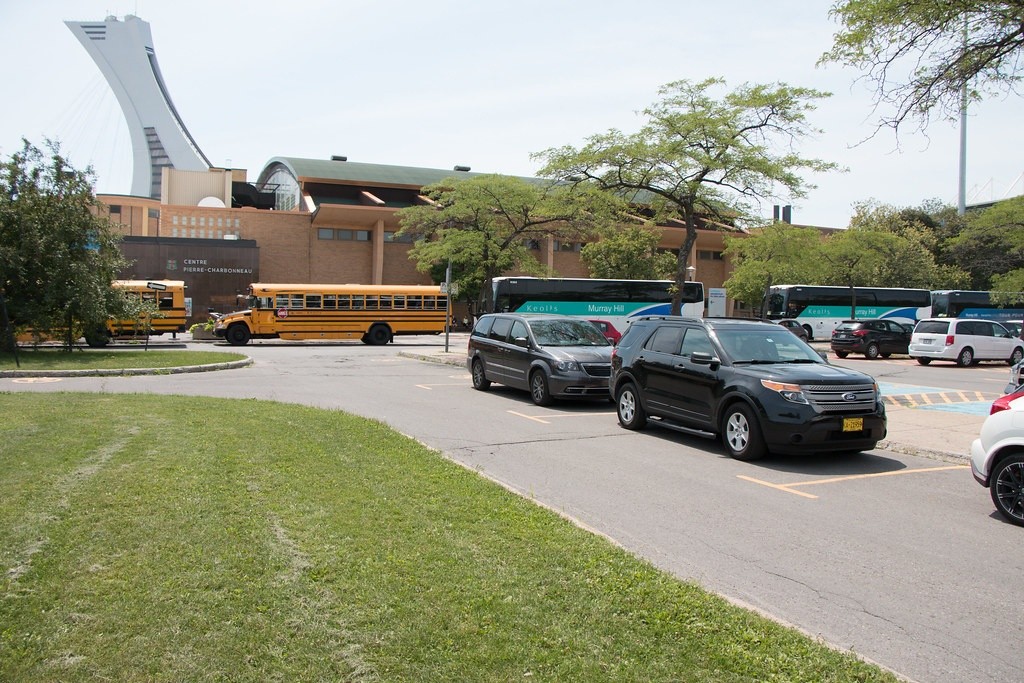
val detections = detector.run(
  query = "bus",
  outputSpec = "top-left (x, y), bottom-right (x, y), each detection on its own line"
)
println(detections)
top-left (467, 277), bottom-right (705, 337)
top-left (931, 290), bottom-right (1024, 321)
top-left (211, 283), bottom-right (452, 347)
top-left (759, 285), bottom-right (933, 340)
top-left (14, 278), bottom-right (187, 348)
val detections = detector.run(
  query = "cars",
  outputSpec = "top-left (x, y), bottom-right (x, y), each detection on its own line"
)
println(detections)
top-left (768, 319), bottom-right (808, 343)
top-left (992, 320), bottom-right (1024, 338)
top-left (970, 390), bottom-right (1024, 528)
top-left (587, 320), bottom-right (622, 344)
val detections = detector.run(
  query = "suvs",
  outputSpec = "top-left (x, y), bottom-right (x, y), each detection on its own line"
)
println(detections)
top-left (466, 312), bottom-right (618, 407)
top-left (830, 318), bottom-right (917, 360)
top-left (608, 316), bottom-right (887, 463)
top-left (908, 318), bottom-right (1024, 369)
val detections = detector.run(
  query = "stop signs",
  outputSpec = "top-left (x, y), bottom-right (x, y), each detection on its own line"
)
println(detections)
top-left (277, 308), bottom-right (287, 318)
